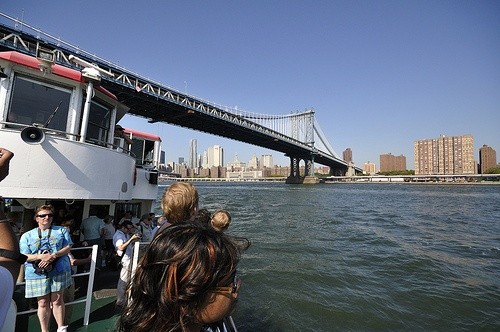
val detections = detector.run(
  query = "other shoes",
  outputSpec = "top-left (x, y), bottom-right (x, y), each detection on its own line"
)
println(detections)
top-left (96, 266), bottom-right (101, 272)
top-left (57, 325), bottom-right (68, 332)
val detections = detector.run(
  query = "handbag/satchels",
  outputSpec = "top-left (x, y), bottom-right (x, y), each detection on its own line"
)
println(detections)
top-left (34, 262), bottom-right (54, 273)
top-left (105, 252), bottom-right (120, 271)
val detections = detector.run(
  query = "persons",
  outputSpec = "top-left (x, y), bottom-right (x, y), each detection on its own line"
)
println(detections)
top-left (0, 147), bottom-right (252, 332)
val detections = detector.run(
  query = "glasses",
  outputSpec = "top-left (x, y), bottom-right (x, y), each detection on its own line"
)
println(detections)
top-left (125, 226), bottom-right (132, 231)
top-left (212, 273), bottom-right (242, 294)
top-left (147, 218), bottom-right (152, 221)
top-left (36, 214), bottom-right (54, 217)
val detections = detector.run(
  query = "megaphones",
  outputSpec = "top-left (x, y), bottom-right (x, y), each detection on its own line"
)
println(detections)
top-left (21, 126), bottom-right (46, 145)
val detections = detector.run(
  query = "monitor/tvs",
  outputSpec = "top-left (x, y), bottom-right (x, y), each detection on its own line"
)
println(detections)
top-left (4, 198), bottom-right (13, 207)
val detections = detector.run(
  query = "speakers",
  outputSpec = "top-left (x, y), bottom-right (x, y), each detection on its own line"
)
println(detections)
top-left (149, 170), bottom-right (158, 184)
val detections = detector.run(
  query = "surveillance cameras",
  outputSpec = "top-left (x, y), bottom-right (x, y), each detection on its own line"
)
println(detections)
top-left (142, 164), bottom-right (156, 169)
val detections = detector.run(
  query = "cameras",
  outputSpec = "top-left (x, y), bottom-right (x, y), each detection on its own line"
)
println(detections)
top-left (37, 249), bottom-right (53, 254)
top-left (135, 234), bottom-right (141, 241)
